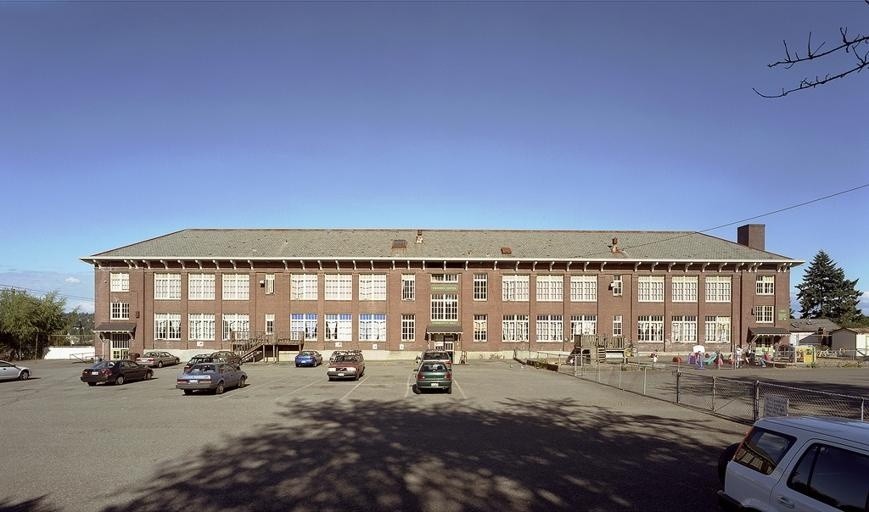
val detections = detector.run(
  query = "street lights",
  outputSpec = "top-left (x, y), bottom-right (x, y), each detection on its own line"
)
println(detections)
top-left (19, 335), bottom-right (23, 361)
top-left (78, 318), bottom-right (83, 344)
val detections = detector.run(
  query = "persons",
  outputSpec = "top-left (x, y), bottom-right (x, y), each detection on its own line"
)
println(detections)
top-left (693, 342), bottom-right (794, 369)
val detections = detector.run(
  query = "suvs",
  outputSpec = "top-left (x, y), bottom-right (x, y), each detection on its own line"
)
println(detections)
top-left (418, 349), bottom-right (452, 373)
top-left (717, 415), bottom-right (869, 511)
top-left (327, 349), bottom-right (365, 380)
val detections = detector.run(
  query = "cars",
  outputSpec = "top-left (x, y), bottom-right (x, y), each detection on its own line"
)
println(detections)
top-left (413, 361), bottom-right (452, 394)
top-left (211, 351), bottom-right (242, 365)
top-left (135, 351), bottom-right (180, 368)
top-left (175, 362), bottom-right (248, 395)
top-left (295, 351), bottom-right (323, 367)
top-left (0, 360), bottom-right (32, 381)
top-left (330, 351), bottom-right (348, 365)
top-left (184, 353), bottom-right (240, 372)
top-left (80, 359), bottom-right (154, 386)
